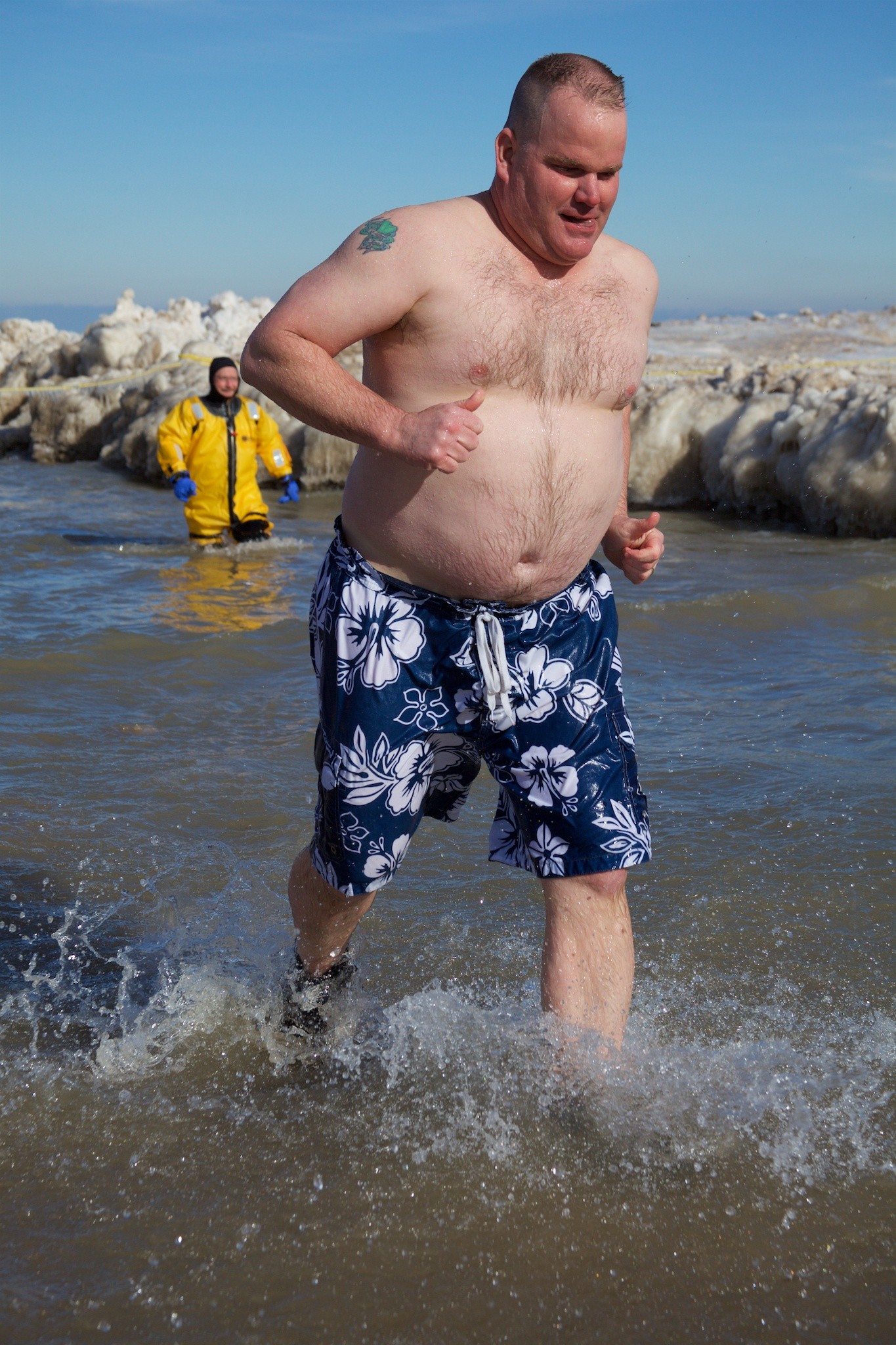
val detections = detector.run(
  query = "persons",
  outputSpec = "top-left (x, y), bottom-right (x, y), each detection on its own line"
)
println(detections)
top-left (156, 357), bottom-right (299, 550)
top-left (239, 53), bottom-right (667, 1053)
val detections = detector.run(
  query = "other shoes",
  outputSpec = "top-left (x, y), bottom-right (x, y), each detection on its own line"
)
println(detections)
top-left (284, 939), bottom-right (355, 1037)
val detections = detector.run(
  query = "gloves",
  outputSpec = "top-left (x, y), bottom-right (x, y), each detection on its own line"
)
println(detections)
top-left (278, 475), bottom-right (300, 503)
top-left (173, 477), bottom-right (197, 502)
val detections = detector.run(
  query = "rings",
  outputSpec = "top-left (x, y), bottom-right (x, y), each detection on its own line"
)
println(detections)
top-left (651, 567), bottom-right (655, 576)
